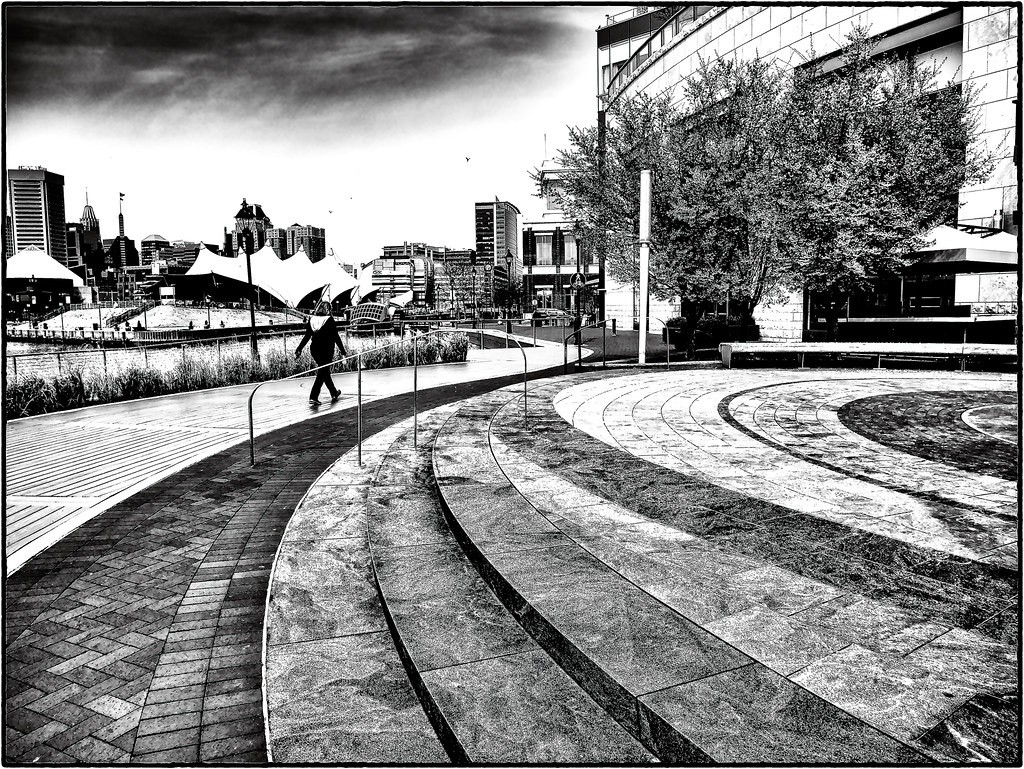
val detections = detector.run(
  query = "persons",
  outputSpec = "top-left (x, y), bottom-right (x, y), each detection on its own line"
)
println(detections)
top-left (296, 301), bottom-right (347, 404)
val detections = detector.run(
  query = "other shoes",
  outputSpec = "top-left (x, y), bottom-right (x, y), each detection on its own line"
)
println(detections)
top-left (331, 390), bottom-right (341, 399)
top-left (309, 398), bottom-right (322, 405)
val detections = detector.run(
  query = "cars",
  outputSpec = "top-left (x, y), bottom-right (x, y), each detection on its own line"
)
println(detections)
top-left (531, 308), bottom-right (575, 328)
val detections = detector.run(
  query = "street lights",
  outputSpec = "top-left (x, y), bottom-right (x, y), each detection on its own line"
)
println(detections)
top-left (471, 265), bottom-right (477, 329)
top-left (205, 295), bottom-right (212, 327)
top-left (570, 219), bottom-right (586, 345)
top-left (58, 303), bottom-right (65, 337)
top-left (505, 249), bottom-right (514, 334)
top-left (97, 301), bottom-right (102, 329)
top-left (142, 297), bottom-right (148, 330)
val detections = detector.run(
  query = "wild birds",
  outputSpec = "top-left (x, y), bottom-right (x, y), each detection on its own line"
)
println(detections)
top-left (328, 156), bottom-right (471, 214)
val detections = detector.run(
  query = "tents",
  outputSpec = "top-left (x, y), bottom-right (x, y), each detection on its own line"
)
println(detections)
top-left (888, 224), bottom-right (1019, 265)
